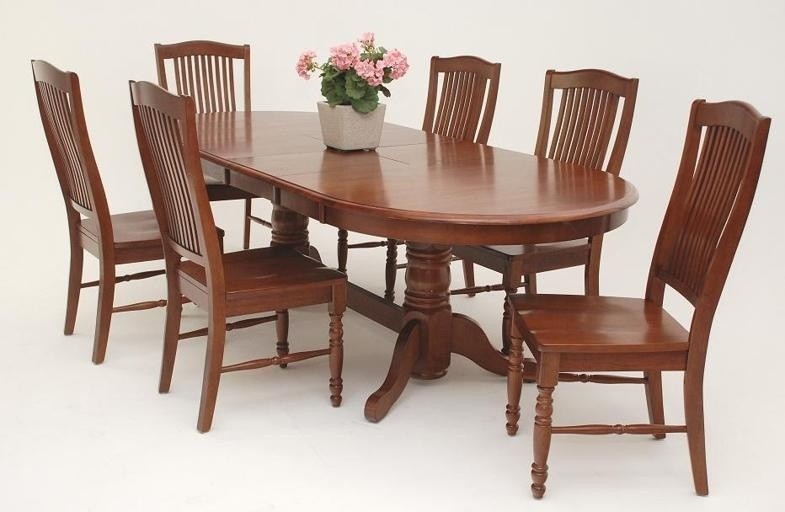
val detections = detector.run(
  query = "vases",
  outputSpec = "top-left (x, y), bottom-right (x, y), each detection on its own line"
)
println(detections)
top-left (317, 101), bottom-right (385, 150)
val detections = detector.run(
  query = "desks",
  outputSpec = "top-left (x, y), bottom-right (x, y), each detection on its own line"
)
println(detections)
top-left (178, 109), bottom-right (638, 423)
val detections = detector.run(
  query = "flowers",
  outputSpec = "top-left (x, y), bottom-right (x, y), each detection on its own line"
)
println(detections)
top-left (296, 32), bottom-right (409, 114)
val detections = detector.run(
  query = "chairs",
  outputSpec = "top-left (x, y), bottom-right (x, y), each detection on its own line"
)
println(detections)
top-left (31, 61), bottom-right (224, 364)
top-left (155, 41), bottom-right (271, 250)
top-left (127, 79), bottom-right (348, 433)
top-left (336, 53), bottom-right (500, 302)
top-left (505, 100), bottom-right (772, 500)
top-left (452, 70), bottom-right (638, 351)
